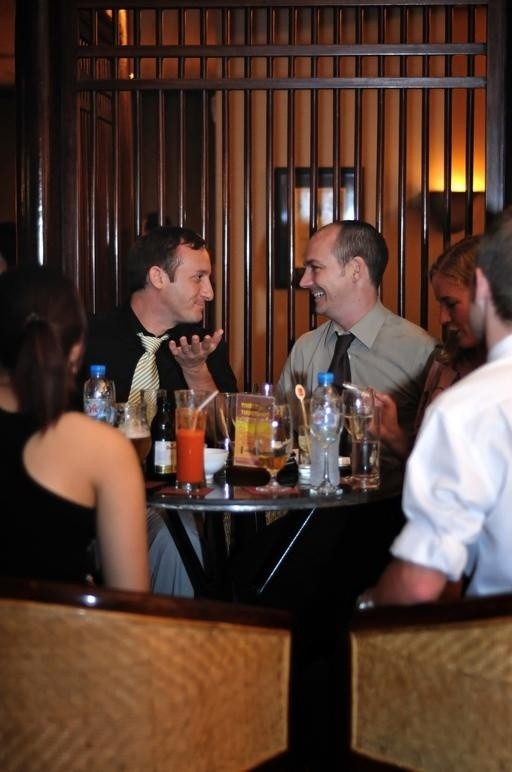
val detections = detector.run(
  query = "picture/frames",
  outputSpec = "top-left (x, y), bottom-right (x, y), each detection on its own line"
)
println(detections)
top-left (274, 167), bottom-right (364, 288)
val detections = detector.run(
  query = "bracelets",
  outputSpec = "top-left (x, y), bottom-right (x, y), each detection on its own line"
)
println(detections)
top-left (353, 585), bottom-right (378, 614)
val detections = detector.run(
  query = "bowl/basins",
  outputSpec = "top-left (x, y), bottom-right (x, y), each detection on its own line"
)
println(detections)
top-left (204, 445), bottom-right (230, 486)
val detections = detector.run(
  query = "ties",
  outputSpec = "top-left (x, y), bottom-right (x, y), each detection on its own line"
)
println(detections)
top-left (124, 332), bottom-right (170, 427)
top-left (327, 332), bottom-right (356, 455)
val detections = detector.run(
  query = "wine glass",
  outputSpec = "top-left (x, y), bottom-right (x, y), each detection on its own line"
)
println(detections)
top-left (252, 404), bottom-right (297, 494)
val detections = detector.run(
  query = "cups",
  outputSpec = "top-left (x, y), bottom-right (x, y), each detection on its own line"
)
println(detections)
top-left (251, 381), bottom-right (277, 398)
top-left (173, 389), bottom-right (212, 493)
top-left (135, 389), bottom-right (166, 425)
top-left (215, 391), bottom-right (236, 439)
top-left (344, 385), bottom-right (385, 490)
top-left (109, 400), bottom-right (153, 463)
top-left (296, 398), bottom-right (321, 489)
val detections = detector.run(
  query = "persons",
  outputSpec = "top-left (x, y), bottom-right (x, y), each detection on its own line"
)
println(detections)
top-left (72, 228), bottom-right (238, 603)
top-left (341, 236), bottom-right (489, 464)
top-left (352, 207), bottom-right (510, 615)
top-left (217, 221), bottom-right (440, 606)
top-left (2, 269), bottom-right (153, 595)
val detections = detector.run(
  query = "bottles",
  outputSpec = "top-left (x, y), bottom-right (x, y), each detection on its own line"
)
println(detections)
top-left (82, 363), bottom-right (117, 427)
top-left (146, 393), bottom-right (176, 483)
top-left (306, 368), bottom-right (343, 500)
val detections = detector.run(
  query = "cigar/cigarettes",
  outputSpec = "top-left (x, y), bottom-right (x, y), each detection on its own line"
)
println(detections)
top-left (341, 382), bottom-right (369, 397)
